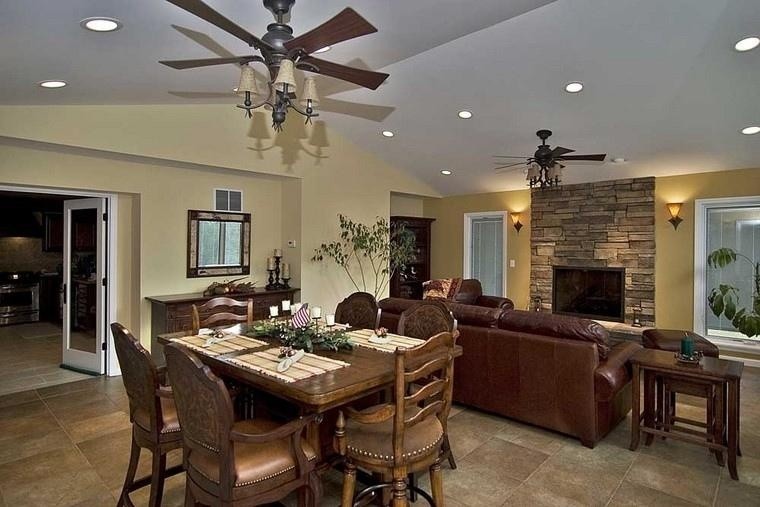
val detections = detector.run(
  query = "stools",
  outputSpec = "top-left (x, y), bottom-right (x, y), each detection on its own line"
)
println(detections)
top-left (641, 329), bottom-right (725, 466)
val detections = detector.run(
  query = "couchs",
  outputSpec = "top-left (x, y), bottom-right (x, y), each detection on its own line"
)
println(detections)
top-left (378, 296), bottom-right (643, 447)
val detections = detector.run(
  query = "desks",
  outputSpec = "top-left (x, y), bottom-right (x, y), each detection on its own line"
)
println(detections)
top-left (628, 348), bottom-right (744, 481)
top-left (146, 288), bottom-right (301, 386)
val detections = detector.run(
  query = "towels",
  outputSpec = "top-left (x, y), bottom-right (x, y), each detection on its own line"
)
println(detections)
top-left (278, 349), bottom-right (304, 373)
top-left (196, 323), bottom-right (240, 336)
top-left (368, 334), bottom-right (392, 344)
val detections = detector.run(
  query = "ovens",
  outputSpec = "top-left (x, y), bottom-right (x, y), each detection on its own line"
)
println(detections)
top-left (0, 283), bottom-right (40, 325)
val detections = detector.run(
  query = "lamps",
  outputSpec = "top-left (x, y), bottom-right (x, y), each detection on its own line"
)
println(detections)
top-left (526, 158), bottom-right (561, 191)
top-left (667, 202), bottom-right (682, 231)
top-left (511, 211), bottom-right (523, 233)
top-left (234, 57), bottom-right (320, 131)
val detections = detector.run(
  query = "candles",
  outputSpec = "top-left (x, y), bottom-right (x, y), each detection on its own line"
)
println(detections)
top-left (267, 248), bottom-right (290, 279)
top-left (268, 300), bottom-right (335, 326)
top-left (681, 332), bottom-right (694, 356)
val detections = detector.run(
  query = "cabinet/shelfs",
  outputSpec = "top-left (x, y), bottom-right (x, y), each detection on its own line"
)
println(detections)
top-left (42, 214), bottom-right (95, 252)
top-left (40, 278), bottom-right (95, 331)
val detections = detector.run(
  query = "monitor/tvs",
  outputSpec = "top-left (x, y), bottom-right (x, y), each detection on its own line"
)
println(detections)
top-left (552, 264), bottom-right (626, 323)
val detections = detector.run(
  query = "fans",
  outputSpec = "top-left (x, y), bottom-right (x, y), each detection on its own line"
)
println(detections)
top-left (157, 1), bottom-right (392, 131)
top-left (492, 130), bottom-right (607, 175)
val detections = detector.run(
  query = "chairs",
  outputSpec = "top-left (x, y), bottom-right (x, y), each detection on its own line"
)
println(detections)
top-left (191, 297), bottom-right (255, 331)
top-left (335, 292), bottom-right (382, 330)
top-left (423, 278), bottom-right (514, 310)
top-left (110, 321), bottom-right (253, 506)
top-left (396, 299), bottom-right (456, 470)
top-left (163, 342), bottom-right (322, 506)
top-left (335, 331), bottom-right (459, 507)
